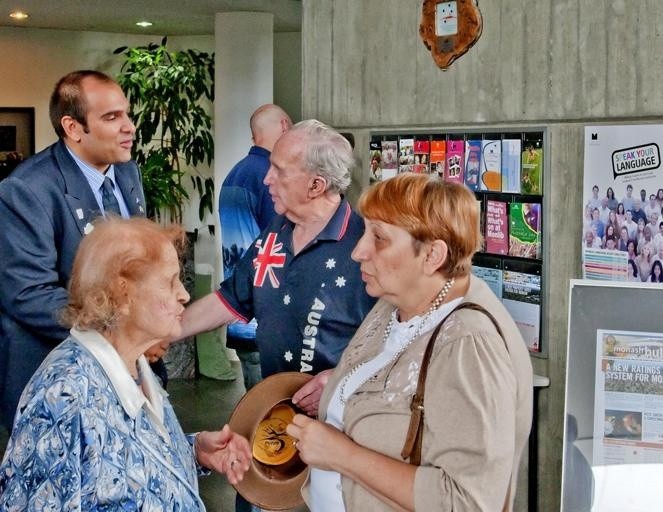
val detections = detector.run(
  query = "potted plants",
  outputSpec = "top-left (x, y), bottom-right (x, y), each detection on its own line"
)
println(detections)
top-left (110, 36), bottom-right (216, 279)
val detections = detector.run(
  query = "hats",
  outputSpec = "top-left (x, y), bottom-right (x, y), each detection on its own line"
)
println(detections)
top-left (223, 370), bottom-right (315, 510)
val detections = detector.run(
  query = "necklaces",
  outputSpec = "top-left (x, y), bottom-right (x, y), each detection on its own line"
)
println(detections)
top-left (339, 277), bottom-right (456, 405)
top-left (132, 360), bottom-right (144, 388)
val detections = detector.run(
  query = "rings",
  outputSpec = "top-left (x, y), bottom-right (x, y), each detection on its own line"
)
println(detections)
top-left (292, 439), bottom-right (300, 448)
top-left (230, 458), bottom-right (239, 468)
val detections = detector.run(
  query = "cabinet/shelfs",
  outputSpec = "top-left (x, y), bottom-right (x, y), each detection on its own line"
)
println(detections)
top-left (369, 124), bottom-right (555, 361)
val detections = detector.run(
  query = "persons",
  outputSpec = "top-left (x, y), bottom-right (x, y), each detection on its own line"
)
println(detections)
top-left (0, 208), bottom-right (253, 511)
top-left (163, 117), bottom-right (380, 512)
top-left (401, 145), bottom-right (410, 155)
top-left (368, 152), bottom-right (380, 178)
top-left (0, 69), bottom-right (165, 452)
top-left (218, 104), bottom-right (296, 385)
top-left (584, 184), bottom-right (663, 282)
top-left (604, 336), bottom-right (625, 357)
top-left (286, 170), bottom-right (535, 512)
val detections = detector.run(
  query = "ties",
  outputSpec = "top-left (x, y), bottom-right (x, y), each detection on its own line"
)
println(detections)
top-left (102, 178), bottom-right (121, 217)
